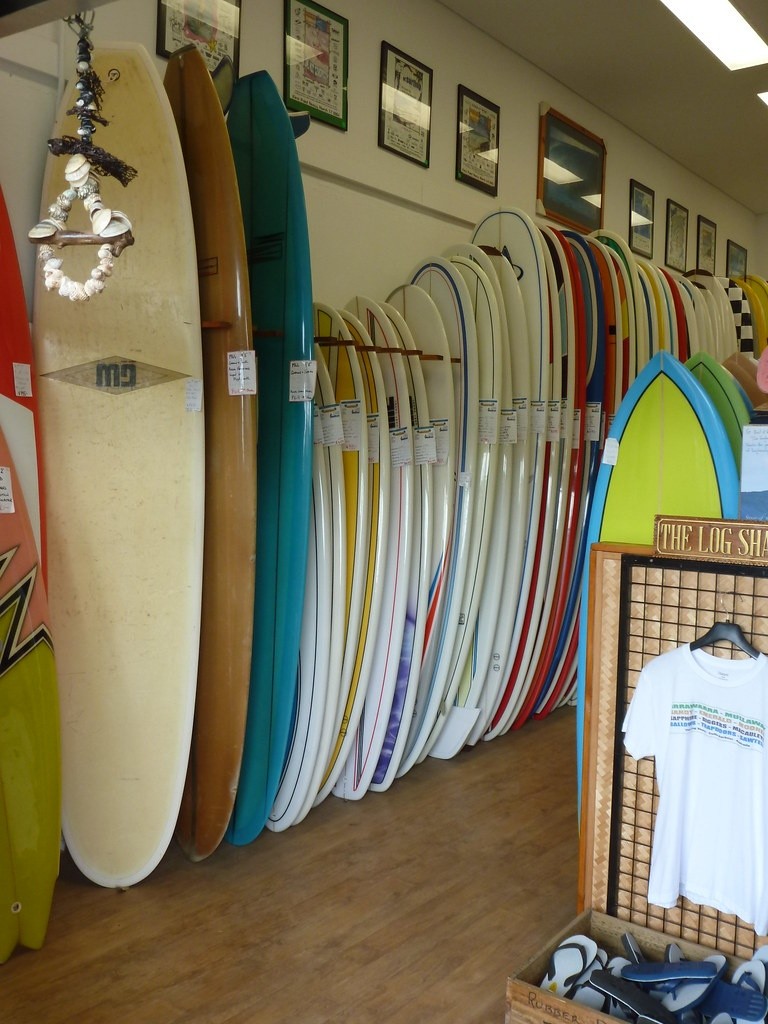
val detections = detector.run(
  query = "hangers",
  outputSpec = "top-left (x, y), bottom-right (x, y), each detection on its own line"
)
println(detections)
top-left (691, 592), bottom-right (761, 663)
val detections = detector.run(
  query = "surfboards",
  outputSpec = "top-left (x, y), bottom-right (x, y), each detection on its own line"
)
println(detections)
top-left (0, 42), bottom-right (767, 968)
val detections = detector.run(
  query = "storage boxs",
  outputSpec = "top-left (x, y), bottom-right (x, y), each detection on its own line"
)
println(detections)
top-left (503, 910), bottom-right (749, 1024)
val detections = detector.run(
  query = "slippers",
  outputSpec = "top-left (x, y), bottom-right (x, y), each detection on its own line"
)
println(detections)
top-left (539, 933), bottom-right (768, 1024)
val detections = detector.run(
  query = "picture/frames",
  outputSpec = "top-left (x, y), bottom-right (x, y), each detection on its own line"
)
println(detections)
top-left (377, 40), bottom-right (433, 168)
top-left (726, 238), bottom-right (747, 281)
top-left (283, 0), bottom-right (349, 131)
top-left (629, 179), bottom-right (655, 259)
top-left (697, 215), bottom-right (717, 276)
top-left (456, 84), bottom-right (501, 196)
top-left (156, 0), bottom-right (242, 81)
top-left (536, 101), bottom-right (608, 235)
top-left (666, 198), bottom-right (689, 273)
